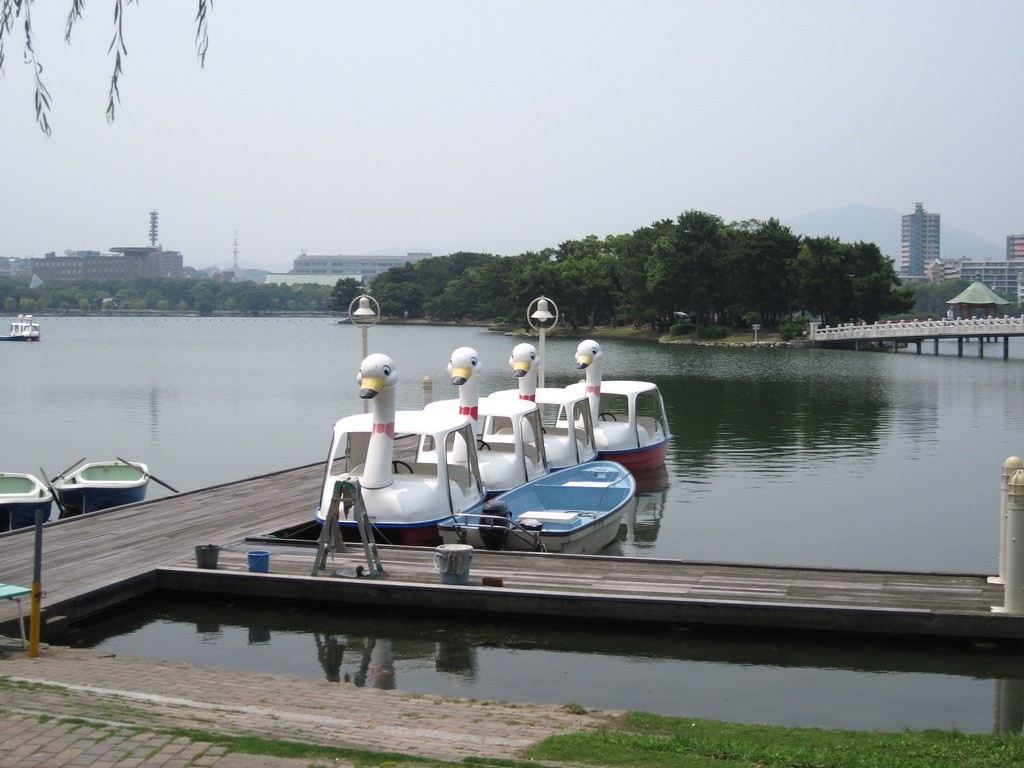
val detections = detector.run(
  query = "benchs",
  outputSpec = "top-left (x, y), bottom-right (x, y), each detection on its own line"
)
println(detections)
top-left (600, 412), bottom-right (664, 438)
top-left (518, 509), bottom-right (580, 529)
top-left (474, 441), bottom-right (544, 471)
top-left (539, 427), bottom-right (592, 456)
top-left (351, 460), bottom-right (479, 500)
top-left (564, 481), bottom-right (615, 488)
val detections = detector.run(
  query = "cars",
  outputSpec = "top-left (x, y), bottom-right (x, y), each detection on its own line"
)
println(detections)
top-left (666, 312), bottom-right (692, 324)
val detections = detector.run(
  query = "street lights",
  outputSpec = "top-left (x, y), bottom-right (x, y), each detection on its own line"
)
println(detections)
top-left (348, 295), bottom-right (381, 415)
top-left (526, 295), bottom-right (559, 421)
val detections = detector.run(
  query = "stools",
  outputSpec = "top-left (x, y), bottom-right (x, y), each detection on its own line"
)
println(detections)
top-left (0, 584), bottom-right (32, 651)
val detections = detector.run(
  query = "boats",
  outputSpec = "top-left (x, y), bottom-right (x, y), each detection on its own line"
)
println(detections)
top-left (0, 313), bottom-right (40, 343)
top-left (0, 471), bottom-right (53, 530)
top-left (437, 459), bottom-right (637, 556)
top-left (553, 339), bottom-right (673, 471)
top-left (54, 460), bottom-right (150, 512)
top-left (313, 343), bottom-right (602, 546)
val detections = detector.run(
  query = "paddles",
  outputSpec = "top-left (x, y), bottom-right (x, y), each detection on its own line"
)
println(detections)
top-left (49, 456), bottom-right (87, 484)
top-left (116, 456), bottom-right (178, 493)
top-left (39, 467), bottom-right (64, 513)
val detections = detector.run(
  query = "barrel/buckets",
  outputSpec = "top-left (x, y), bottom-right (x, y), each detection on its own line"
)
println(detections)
top-left (247, 551), bottom-right (270, 573)
top-left (195, 544), bottom-right (223, 569)
top-left (433, 544), bottom-right (473, 585)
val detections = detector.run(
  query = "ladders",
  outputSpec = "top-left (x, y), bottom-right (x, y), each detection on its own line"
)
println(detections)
top-left (310, 474), bottom-right (383, 579)
